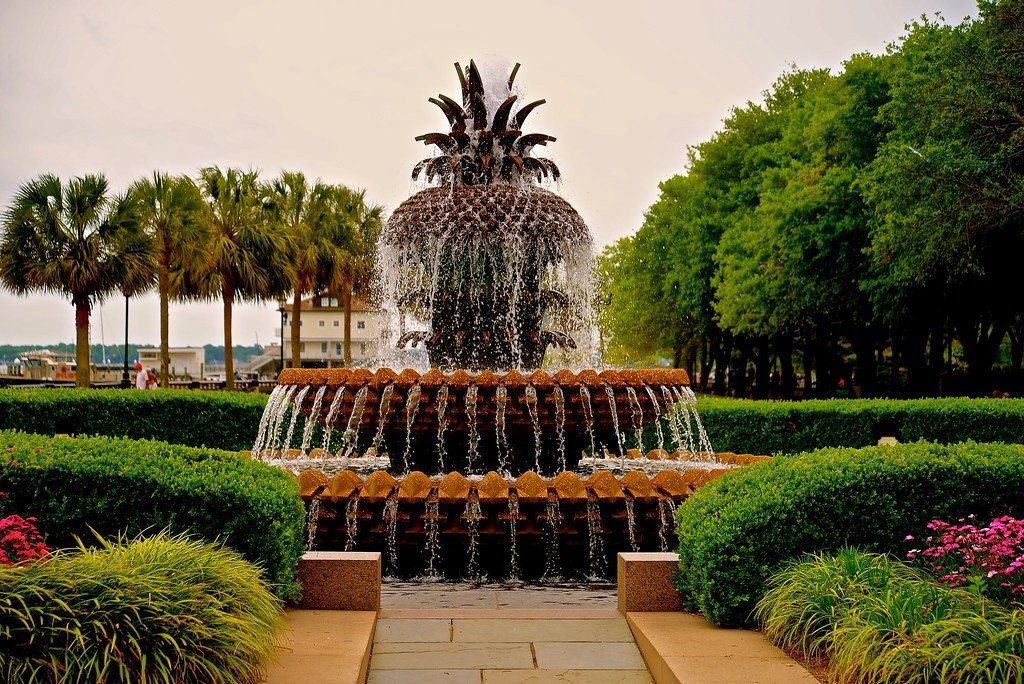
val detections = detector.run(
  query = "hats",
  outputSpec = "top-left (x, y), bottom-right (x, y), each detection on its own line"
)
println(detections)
top-left (134, 363), bottom-right (142, 370)
top-left (237, 375), bottom-right (241, 378)
top-left (151, 368), bottom-right (156, 373)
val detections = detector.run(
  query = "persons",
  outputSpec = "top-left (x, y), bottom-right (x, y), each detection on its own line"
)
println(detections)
top-left (710, 362), bottom-right (798, 397)
top-left (261, 372), bottom-right (267, 381)
top-left (233, 370), bottom-right (244, 380)
top-left (133, 362), bottom-right (159, 389)
